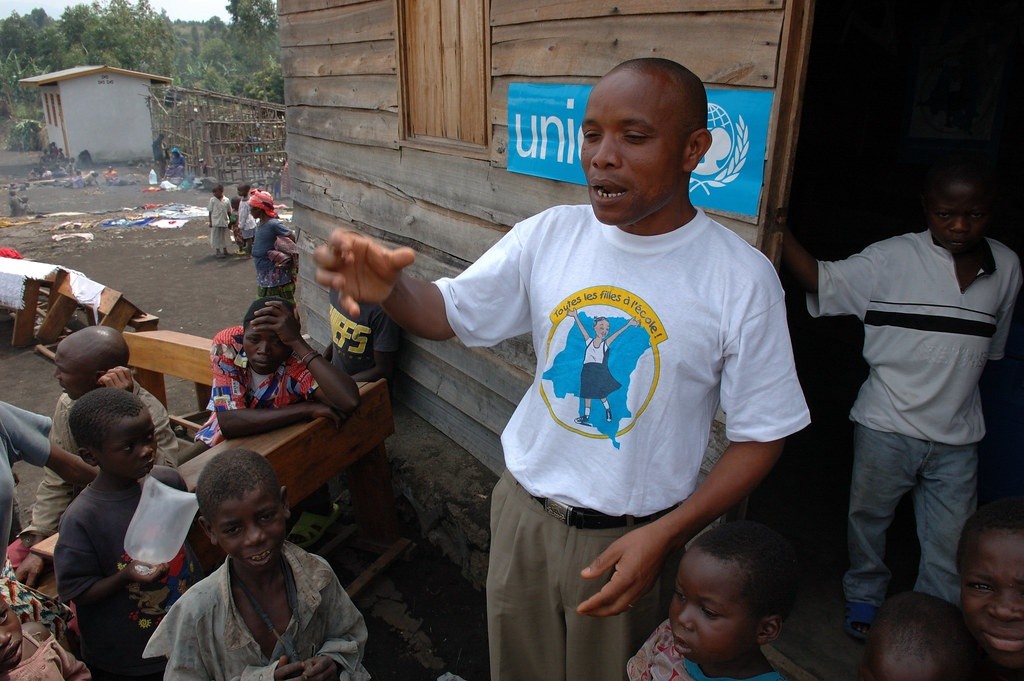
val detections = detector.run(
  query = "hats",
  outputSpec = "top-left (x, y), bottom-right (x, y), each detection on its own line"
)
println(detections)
top-left (171, 148), bottom-right (181, 156)
top-left (248, 189), bottom-right (278, 218)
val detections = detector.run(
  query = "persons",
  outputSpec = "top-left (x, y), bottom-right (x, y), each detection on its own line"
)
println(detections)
top-left (859, 590), bottom-right (977, 681)
top-left (309, 57), bottom-right (813, 681)
top-left (955, 496), bottom-right (1024, 681)
top-left (783, 153), bottom-right (1023, 639)
top-left (627, 520), bottom-right (800, 681)
top-left (0, 134), bottom-right (404, 681)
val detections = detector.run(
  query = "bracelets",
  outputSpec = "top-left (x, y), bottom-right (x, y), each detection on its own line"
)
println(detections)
top-left (305, 353), bottom-right (323, 370)
top-left (301, 351), bottom-right (319, 364)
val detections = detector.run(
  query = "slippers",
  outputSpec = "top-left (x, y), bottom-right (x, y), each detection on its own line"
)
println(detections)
top-left (844, 601), bottom-right (878, 642)
top-left (288, 502), bottom-right (339, 548)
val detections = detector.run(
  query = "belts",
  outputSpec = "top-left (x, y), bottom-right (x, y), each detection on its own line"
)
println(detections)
top-left (533, 496), bottom-right (679, 530)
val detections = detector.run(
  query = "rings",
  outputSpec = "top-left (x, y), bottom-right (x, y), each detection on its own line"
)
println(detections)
top-left (626, 603), bottom-right (636, 610)
